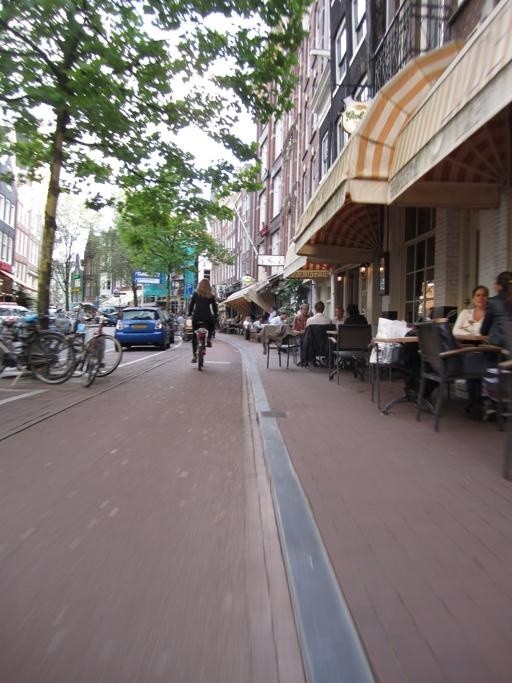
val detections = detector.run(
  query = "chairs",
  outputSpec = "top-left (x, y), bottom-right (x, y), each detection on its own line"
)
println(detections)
top-left (225, 318), bottom-right (371, 385)
top-left (369, 317), bottom-right (511, 430)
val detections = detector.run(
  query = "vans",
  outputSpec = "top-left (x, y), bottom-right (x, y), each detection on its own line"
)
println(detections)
top-left (93, 304), bottom-right (117, 326)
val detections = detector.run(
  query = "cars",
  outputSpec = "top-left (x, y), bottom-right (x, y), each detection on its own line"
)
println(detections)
top-left (114, 305), bottom-right (171, 349)
top-left (2, 300), bottom-right (82, 336)
top-left (162, 309), bottom-right (174, 342)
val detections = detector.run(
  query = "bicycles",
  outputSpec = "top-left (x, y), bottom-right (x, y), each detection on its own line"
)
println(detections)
top-left (0, 318), bottom-right (124, 389)
top-left (185, 310), bottom-right (218, 371)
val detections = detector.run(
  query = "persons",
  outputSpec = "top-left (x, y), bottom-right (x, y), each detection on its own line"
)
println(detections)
top-left (481, 271), bottom-right (512, 422)
top-left (306, 301), bottom-right (332, 327)
top-left (188, 279), bottom-right (218, 363)
top-left (344, 304), bottom-right (367, 324)
top-left (242, 307), bottom-right (288, 339)
top-left (292, 304), bottom-right (310, 365)
top-left (452, 286), bottom-right (489, 335)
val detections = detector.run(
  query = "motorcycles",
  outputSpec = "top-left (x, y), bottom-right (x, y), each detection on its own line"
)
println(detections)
top-left (180, 315), bottom-right (194, 341)
